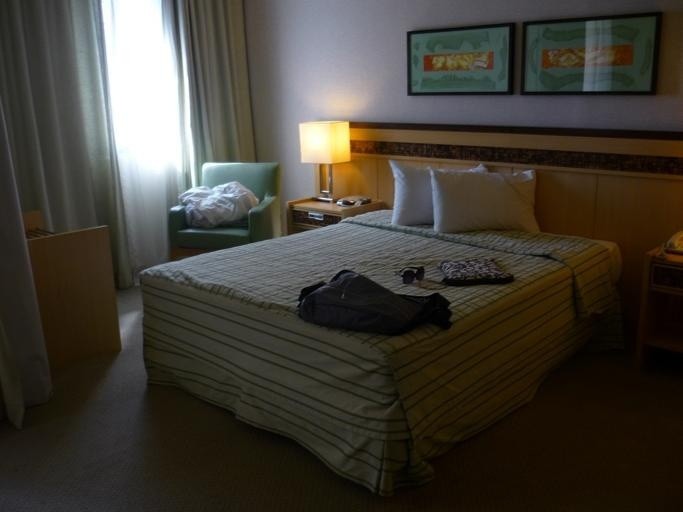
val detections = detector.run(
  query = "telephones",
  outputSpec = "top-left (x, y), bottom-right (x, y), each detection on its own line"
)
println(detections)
top-left (665, 230), bottom-right (683, 252)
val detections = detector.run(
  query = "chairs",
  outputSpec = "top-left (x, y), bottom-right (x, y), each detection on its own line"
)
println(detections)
top-left (166, 162), bottom-right (286, 262)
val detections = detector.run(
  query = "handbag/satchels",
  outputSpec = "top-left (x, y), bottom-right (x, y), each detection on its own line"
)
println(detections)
top-left (441, 256), bottom-right (515, 286)
top-left (295, 269), bottom-right (452, 333)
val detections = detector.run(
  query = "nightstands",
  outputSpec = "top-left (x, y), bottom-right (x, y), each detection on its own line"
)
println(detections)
top-left (284, 195), bottom-right (384, 236)
top-left (629, 245), bottom-right (683, 378)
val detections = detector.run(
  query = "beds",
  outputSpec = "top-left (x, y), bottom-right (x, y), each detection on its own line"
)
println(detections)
top-left (136, 207), bottom-right (625, 500)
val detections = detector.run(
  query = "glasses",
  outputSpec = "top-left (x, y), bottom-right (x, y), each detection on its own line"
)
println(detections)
top-left (394, 266), bottom-right (425, 285)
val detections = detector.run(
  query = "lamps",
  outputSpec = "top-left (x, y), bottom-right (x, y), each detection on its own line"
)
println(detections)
top-left (295, 119), bottom-right (353, 203)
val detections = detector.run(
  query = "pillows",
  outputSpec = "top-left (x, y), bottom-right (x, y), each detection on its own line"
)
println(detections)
top-left (424, 163), bottom-right (546, 237)
top-left (384, 157), bottom-right (488, 229)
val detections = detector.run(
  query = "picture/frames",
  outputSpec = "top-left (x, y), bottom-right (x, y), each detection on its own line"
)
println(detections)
top-left (519, 9), bottom-right (665, 100)
top-left (406, 21), bottom-right (517, 97)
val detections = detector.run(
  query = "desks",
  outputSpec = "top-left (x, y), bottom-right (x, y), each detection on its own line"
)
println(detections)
top-left (19, 208), bottom-right (122, 362)
